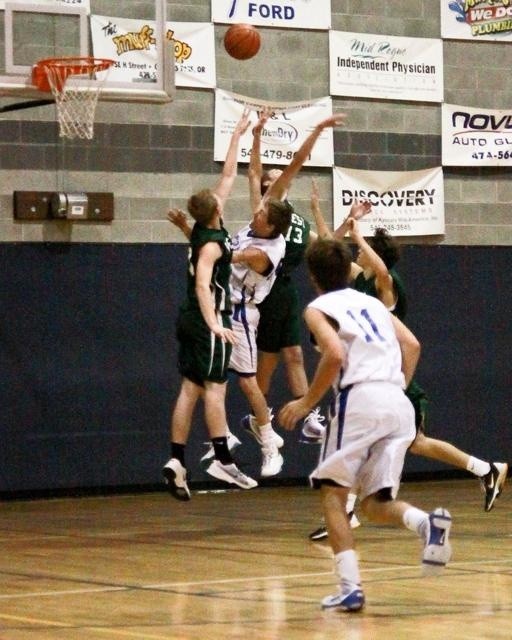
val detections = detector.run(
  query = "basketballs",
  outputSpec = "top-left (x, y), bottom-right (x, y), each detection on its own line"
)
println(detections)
top-left (225, 24), bottom-right (260, 59)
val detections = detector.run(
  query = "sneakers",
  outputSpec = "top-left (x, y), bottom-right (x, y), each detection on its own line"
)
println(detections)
top-left (308, 512), bottom-right (361, 541)
top-left (422, 507), bottom-right (452, 576)
top-left (161, 409), bottom-right (325, 501)
top-left (480, 461), bottom-right (509, 512)
top-left (322, 585), bottom-right (364, 612)
